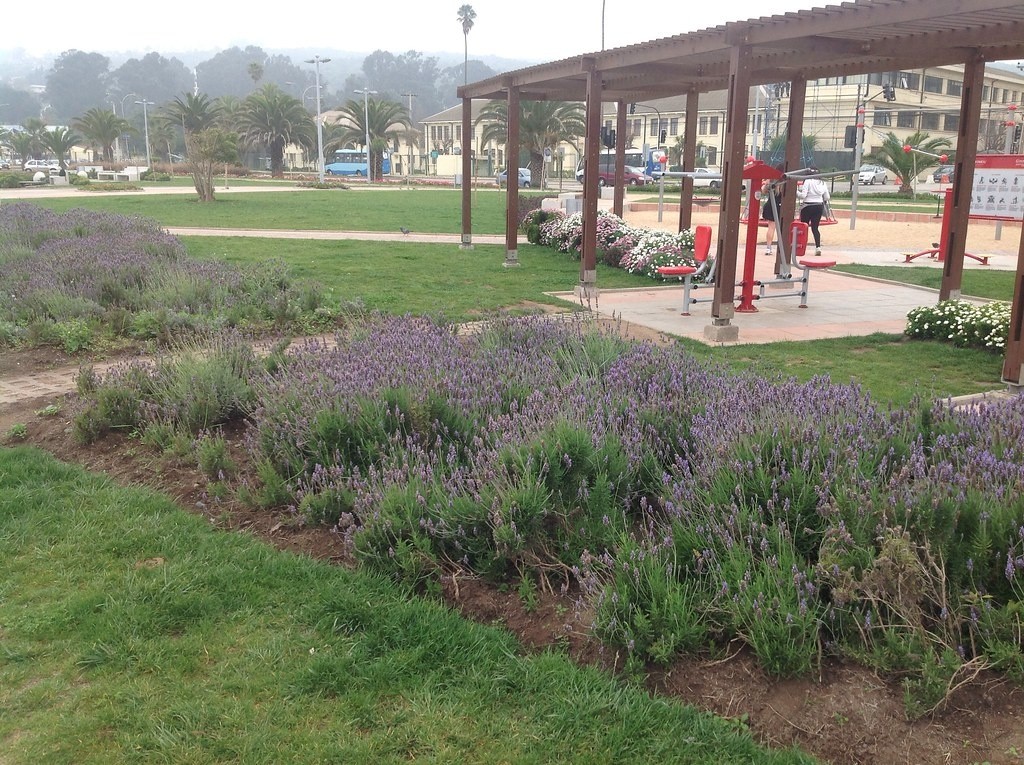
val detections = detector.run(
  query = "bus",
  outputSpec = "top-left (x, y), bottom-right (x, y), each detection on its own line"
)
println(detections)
top-left (575, 148), bottom-right (643, 185)
top-left (324, 149), bottom-right (390, 176)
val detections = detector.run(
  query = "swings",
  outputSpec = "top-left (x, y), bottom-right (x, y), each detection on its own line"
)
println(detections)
top-left (739, 122), bottom-right (839, 226)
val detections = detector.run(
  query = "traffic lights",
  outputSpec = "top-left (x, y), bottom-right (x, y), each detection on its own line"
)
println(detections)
top-left (883, 83), bottom-right (892, 100)
top-left (1014, 125), bottom-right (1022, 144)
top-left (661, 129), bottom-right (666, 143)
top-left (630, 102), bottom-right (636, 114)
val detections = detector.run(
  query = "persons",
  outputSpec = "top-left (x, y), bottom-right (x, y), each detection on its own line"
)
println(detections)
top-left (761, 163), bottom-right (785, 255)
top-left (796, 168), bottom-right (831, 256)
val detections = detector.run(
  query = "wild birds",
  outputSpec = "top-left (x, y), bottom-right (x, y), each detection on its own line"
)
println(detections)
top-left (399, 227), bottom-right (413, 237)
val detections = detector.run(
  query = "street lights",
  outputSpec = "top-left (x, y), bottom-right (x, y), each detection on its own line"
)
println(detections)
top-left (353, 87), bottom-right (378, 183)
top-left (302, 85), bottom-right (323, 108)
top-left (303, 54), bottom-right (331, 183)
top-left (134, 98), bottom-right (156, 168)
top-left (106, 93), bottom-right (136, 164)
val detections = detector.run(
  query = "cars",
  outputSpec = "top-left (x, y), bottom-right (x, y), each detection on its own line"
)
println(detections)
top-left (48, 159), bottom-right (70, 169)
top-left (597, 164), bottom-right (655, 187)
top-left (495, 168), bottom-right (548, 187)
top-left (931, 165), bottom-right (955, 183)
top-left (693, 168), bottom-right (722, 190)
top-left (852, 164), bottom-right (888, 185)
top-left (0, 159), bottom-right (10, 170)
top-left (24, 159), bottom-right (62, 172)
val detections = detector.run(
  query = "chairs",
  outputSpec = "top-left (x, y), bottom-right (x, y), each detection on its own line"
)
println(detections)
top-left (658, 226), bottom-right (712, 315)
top-left (789, 222), bottom-right (836, 309)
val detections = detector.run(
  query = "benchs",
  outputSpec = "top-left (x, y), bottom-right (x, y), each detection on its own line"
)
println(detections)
top-left (49, 176), bottom-right (65, 185)
top-left (97, 172), bottom-right (137, 181)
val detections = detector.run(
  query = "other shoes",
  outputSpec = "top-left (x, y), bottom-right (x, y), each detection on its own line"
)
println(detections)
top-left (765, 248), bottom-right (772, 255)
top-left (815, 247), bottom-right (821, 256)
